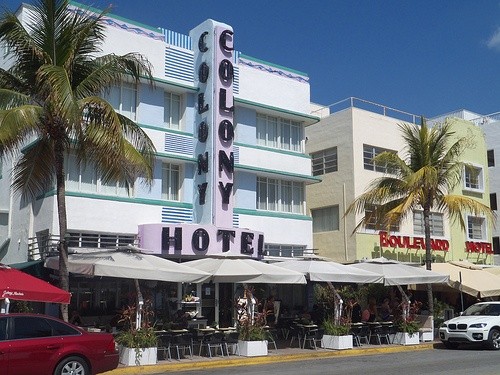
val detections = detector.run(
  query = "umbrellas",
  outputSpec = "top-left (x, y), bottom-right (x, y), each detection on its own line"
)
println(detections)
top-left (0, 263), bottom-right (71, 309)
top-left (422, 260), bottom-right (500, 299)
top-left (43, 245), bottom-right (307, 285)
top-left (270, 256), bottom-right (451, 287)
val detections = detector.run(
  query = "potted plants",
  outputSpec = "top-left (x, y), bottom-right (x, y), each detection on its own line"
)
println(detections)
top-left (236, 308), bottom-right (270, 356)
top-left (389, 300), bottom-right (424, 345)
top-left (315, 285), bottom-right (365, 350)
top-left (117, 299), bottom-right (157, 366)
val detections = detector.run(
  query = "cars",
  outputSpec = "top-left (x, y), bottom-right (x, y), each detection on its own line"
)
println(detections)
top-left (438, 301), bottom-right (500, 350)
top-left (1, 313), bottom-right (121, 375)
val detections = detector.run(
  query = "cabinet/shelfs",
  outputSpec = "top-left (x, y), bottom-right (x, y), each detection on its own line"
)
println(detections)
top-left (187, 319), bottom-right (209, 328)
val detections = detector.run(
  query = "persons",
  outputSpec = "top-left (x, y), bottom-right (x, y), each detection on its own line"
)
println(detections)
top-left (381, 296), bottom-right (393, 321)
top-left (362, 303), bottom-right (377, 322)
top-left (265, 295), bottom-right (276, 328)
top-left (310, 304), bottom-right (322, 322)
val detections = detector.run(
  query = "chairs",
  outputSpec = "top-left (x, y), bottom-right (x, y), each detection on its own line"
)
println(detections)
top-left (264, 325), bottom-right (323, 352)
top-left (350, 321), bottom-right (391, 348)
top-left (157, 332), bottom-right (240, 361)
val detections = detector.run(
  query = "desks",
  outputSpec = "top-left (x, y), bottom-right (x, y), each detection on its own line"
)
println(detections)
top-left (157, 327), bottom-right (236, 332)
